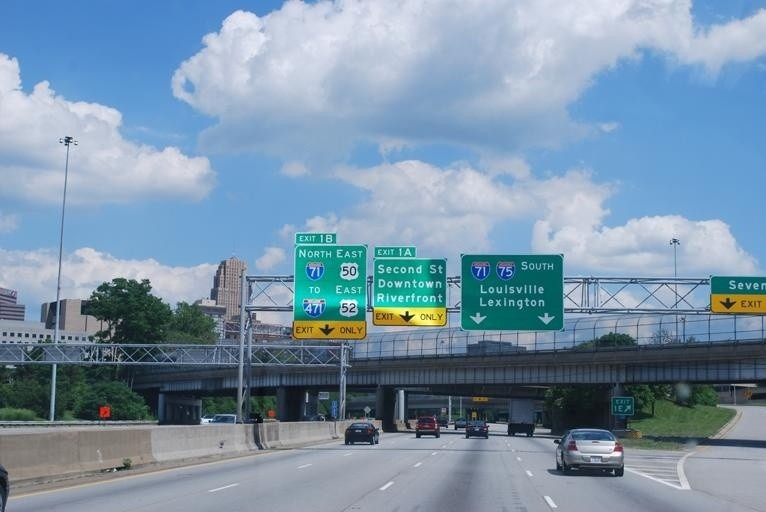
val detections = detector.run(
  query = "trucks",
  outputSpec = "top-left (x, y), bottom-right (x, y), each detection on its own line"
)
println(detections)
top-left (507, 398), bottom-right (536, 436)
top-left (208, 413), bottom-right (281, 423)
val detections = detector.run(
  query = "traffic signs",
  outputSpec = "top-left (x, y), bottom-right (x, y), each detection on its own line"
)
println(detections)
top-left (298, 233), bottom-right (336, 244)
top-left (710, 294), bottom-right (766, 315)
top-left (710, 275), bottom-right (766, 294)
top-left (372, 256), bottom-right (446, 307)
top-left (373, 306), bottom-right (448, 328)
top-left (374, 247), bottom-right (416, 256)
top-left (293, 321), bottom-right (367, 340)
top-left (462, 255), bottom-right (563, 331)
top-left (612, 396), bottom-right (634, 414)
top-left (294, 245), bottom-right (367, 321)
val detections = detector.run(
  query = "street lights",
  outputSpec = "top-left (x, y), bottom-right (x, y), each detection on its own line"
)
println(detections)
top-left (438, 339), bottom-right (445, 355)
top-left (670, 237), bottom-right (681, 341)
top-left (47, 134), bottom-right (78, 421)
top-left (680, 317), bottom-right (688, 342)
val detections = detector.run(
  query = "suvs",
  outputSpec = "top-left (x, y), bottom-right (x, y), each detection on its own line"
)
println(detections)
top-left (415, 416), bottom-right (441, 439)
top-left (436, 416), bottom-right (449, 428)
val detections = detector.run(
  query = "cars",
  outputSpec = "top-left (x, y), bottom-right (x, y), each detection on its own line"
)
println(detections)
top-left (200, 413), bottom-right (215, 423)
top-left (553, 428), bottom-right (625, 476)
top-left (0, 464), bottom-right (10, 512)
top-left (296, 413), bottom-right (336, 421)
top-left (454, 417), bottom-right (469, 429)
top-left (465, 419), bottom-right (490, 438)
top-left (345, 422), bottom-right (380, 445)
